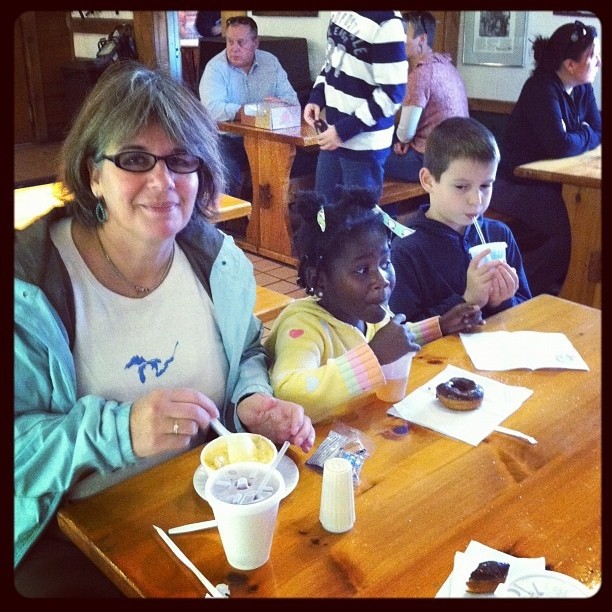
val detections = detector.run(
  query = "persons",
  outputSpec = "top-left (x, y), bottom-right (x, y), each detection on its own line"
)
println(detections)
top-left (489, 21), bottom-right (603, 295)
top-left (262, 185), bottom-right (486, 422)
top-left (199, 17), bottom-right (302, 192)
top-left (281, 10), bottom-right (409, 266)
top-left (383, 10), bottom-right (472, 183)
top-left (13, 59), bottom-right (317, 601)
top-left (385, 117), bottom-right (532, 332)
top-left (194, 9), bottom-right (222, 39)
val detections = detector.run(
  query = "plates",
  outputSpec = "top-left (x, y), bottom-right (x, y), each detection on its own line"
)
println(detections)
top-left (193, 454), bottom-right (300, 501)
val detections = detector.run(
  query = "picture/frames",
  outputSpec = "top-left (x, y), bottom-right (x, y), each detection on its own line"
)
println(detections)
top-left (462, 10), bottom-right (529, 69)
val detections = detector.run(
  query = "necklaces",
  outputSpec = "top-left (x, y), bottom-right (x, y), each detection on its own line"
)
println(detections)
top-left (92, 223), bottom-right (175, 294)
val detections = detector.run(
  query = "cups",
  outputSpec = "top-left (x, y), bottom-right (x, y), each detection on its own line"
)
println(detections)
top-left (374, 352), bottom-right (416, 403)
top-left (468, 241), bottom-right (509, 270)
top-left (205, 461), bottom-right (286, 571)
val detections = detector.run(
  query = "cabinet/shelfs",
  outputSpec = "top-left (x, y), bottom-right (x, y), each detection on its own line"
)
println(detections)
top-left (198, 37), bottom-right (313, 93)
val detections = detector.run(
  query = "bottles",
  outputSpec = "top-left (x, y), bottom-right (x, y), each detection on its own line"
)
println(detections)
top-left (319, 458), bottom-right (356, 534)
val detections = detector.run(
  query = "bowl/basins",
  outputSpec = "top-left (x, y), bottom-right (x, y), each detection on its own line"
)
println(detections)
top-left (200, 432), bottom-right (278, 477)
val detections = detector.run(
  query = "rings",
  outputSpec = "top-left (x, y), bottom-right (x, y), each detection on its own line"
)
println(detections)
top-left (173, 419), bottom-right (179, 435)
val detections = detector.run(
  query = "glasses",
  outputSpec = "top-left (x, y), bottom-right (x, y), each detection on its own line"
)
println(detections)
top-left (571, 21), bottom-right (588, 44)
top-left (226, 17), bottom-right (250, 24)
top-left (103, 152), bottom-right (204, 174)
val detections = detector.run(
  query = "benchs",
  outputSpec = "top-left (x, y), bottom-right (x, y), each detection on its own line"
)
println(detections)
top-left (249, 283), bottom-right (295, 325)
top-left (377, 179), bottom-right (428, 207)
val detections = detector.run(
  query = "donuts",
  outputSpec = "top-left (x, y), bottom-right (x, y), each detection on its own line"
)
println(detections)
top-left (434, 375), bottom-right (484, 411)
top-left (466, 559), bottom-right (510, 593)
